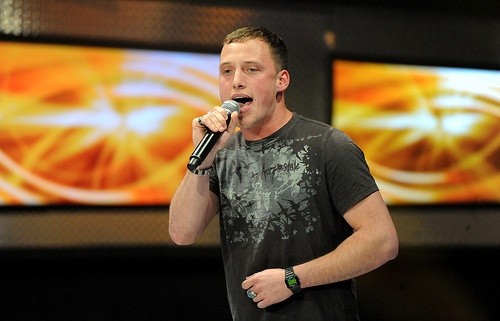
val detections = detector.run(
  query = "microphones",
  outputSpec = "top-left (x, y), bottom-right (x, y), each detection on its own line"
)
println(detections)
top-left (187, 100), bottom-right (240, 171)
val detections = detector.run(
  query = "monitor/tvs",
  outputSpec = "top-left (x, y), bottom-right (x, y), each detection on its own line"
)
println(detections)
top-left (324, 50), bottom-right (500, 209)
top-left (0, 33), bottom-right (241, 212)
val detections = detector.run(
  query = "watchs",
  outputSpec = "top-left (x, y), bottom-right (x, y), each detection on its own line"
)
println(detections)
top-left (285, 267), bottom-right (302, 293)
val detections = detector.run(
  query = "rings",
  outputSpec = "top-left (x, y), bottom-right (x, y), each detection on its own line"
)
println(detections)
top-left (247, 291), bottom-right (258, 299)
top-left (198, 117), bottom-right (204, 126)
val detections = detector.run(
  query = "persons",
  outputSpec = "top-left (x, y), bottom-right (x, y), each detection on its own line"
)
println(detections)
top-left (168, 25), bottom-right (402, 321)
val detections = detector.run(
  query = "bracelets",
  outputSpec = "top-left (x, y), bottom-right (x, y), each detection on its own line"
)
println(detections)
top-left (188, 166), bottom-right (214, 177)
